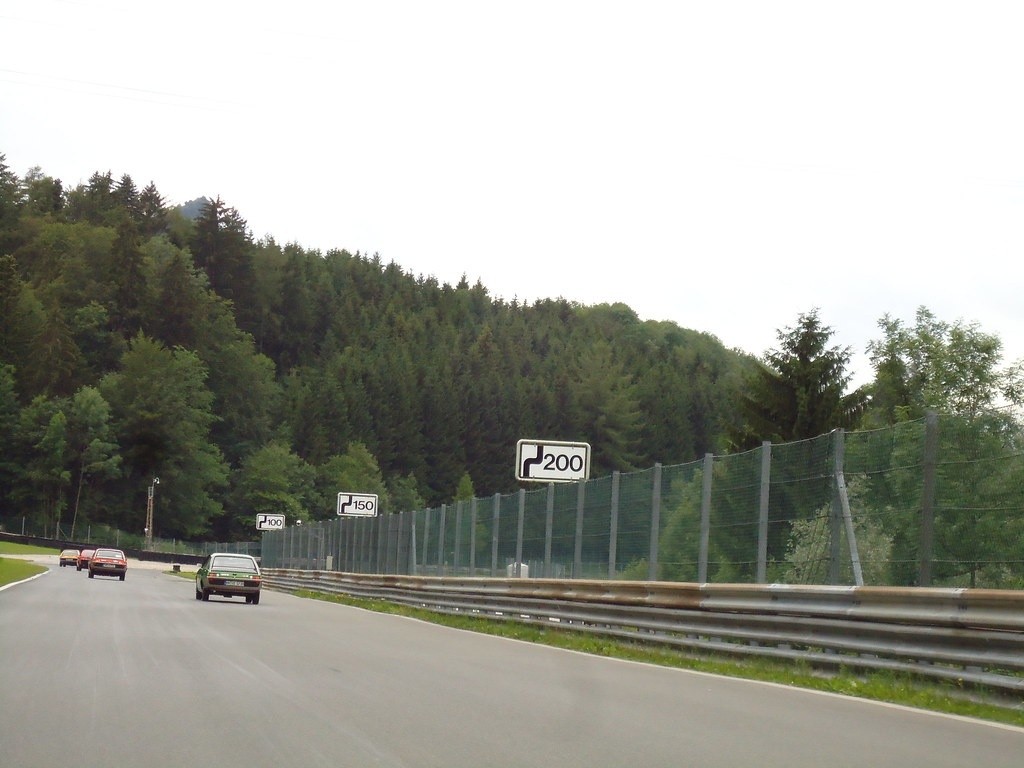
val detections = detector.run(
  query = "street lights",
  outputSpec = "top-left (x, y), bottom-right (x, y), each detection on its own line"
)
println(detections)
top-left (144, 478), bottom-right (160, 551)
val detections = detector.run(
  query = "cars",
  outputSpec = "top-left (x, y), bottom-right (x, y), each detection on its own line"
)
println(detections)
top-left (195, 553), bottom-right (262, 604)
top-left (87, 548), bottom-right (127, 580)
top-left (59, 548), bottom-right (81, 567)
top-left (74, 549), bottom-right (96, 571)
